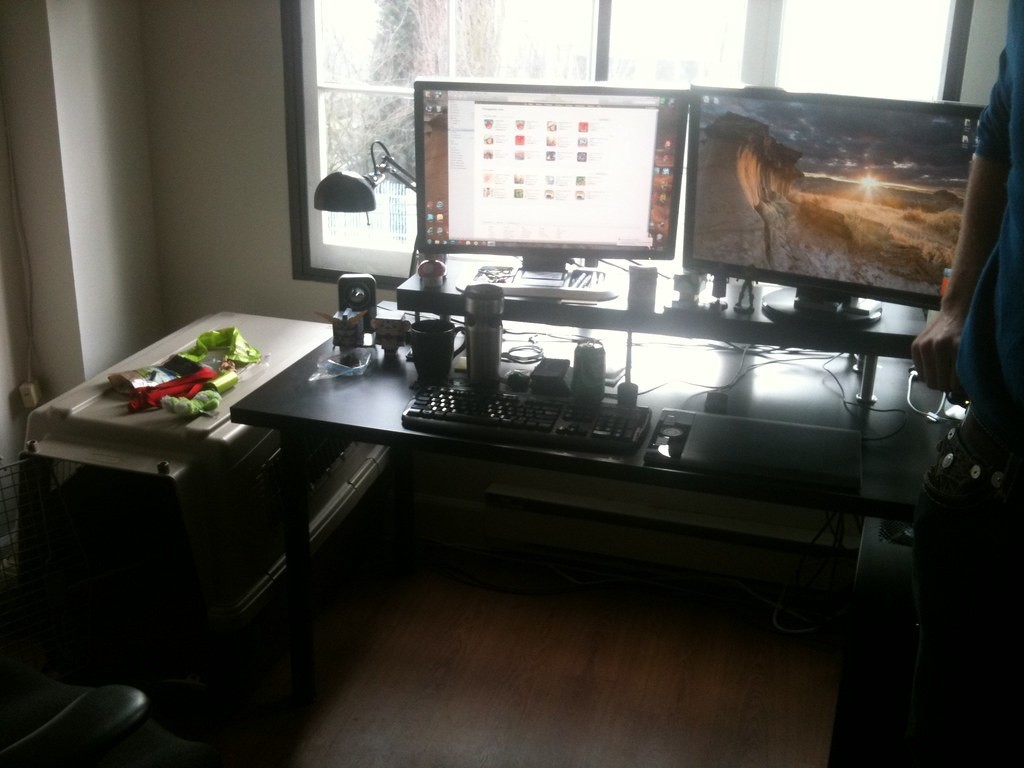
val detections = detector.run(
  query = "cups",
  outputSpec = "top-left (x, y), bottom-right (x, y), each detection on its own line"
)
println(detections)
top-left (412, 319), bottom-right (466, 383)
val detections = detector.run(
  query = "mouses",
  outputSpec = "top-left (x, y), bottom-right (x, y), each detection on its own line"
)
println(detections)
top-left (502, 370), bottom-right (530, 392)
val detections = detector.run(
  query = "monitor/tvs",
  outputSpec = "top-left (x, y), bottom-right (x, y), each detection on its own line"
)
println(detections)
top-left (414, 80), bottom-right (690, 301)
top-left (682, 84), bottom-right (987, 328)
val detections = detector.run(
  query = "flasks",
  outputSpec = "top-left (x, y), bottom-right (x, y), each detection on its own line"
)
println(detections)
top-left (466, 284), bottom-right (504, 384)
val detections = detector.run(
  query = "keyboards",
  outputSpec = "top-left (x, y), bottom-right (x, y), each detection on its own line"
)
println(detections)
top-left (401, 385), bottom-right (652, 455)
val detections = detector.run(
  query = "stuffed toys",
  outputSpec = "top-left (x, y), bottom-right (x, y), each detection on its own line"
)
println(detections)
top-left (160, 390), bottom-right (221, 417)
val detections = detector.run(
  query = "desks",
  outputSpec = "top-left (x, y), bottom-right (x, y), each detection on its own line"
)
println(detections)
top-left (231, 266), bottom-right (959, 720)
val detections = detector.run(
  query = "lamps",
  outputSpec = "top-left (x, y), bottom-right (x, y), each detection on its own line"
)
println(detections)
top-left (312, 141), bottom-right (419, 277)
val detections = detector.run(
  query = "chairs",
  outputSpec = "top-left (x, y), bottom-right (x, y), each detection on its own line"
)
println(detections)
top-left (0, 655), bottom-right (226, 768)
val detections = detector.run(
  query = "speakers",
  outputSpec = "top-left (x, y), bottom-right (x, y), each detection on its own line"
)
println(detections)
top-left (336, 274), bottom-right (377, 333)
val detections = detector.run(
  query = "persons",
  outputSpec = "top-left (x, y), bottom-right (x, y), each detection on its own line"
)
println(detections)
top-left (908, 0), bottom-right (1023, 768)
